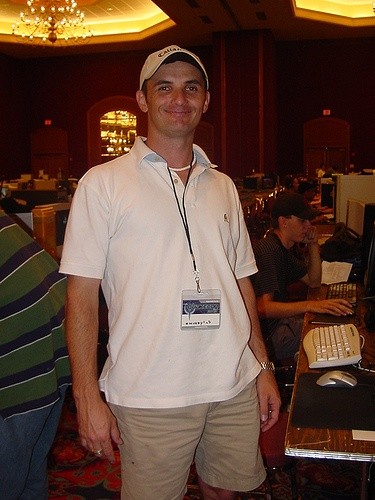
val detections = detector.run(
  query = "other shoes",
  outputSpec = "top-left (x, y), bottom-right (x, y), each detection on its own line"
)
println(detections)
top-left (297, 463), bottom-right (356, 492)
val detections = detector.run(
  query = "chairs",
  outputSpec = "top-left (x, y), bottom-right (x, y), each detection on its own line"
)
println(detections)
top-left (242, 184), bottom-right (286, 234)
top-left (259, 411), bottom-right (298, 500)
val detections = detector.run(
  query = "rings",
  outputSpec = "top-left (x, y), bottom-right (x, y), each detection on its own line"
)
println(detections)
top-left (95, 449), bottom-right (103, 455)
top-left (268, 410), bottom-right (272, 413)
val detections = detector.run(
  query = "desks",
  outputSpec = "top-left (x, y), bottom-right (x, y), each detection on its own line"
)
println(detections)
top-left (283, 202), bottom-right (375, 462)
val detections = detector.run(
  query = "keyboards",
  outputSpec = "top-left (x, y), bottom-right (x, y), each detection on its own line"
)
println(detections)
top-left (303, 323), bottom-right (362, 369)
top-left (328, 282), bottom-right (357, 306)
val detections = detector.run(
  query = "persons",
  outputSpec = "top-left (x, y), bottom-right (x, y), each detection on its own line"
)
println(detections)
top-left (250, 176), bottom-right (354, 371)
top-left (318, 163), bottom-right (339, 178)
top-left (0, 221), bottom-right (71, 500)
top-left (59, 43), bottom-right (281, 499)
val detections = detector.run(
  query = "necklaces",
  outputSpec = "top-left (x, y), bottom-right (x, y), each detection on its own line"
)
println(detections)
top-left (168, 149), bottom-right (196, 171)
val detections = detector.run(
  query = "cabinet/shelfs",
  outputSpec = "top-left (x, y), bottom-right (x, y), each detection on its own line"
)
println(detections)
top-left (100, 135), bottom-right (133, 157)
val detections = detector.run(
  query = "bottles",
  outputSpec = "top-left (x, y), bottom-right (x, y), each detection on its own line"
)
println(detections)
top-left (57, 168), bottom-right (63, 189)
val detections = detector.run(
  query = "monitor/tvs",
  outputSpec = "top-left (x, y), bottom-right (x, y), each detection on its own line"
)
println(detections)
top-left (363, 222), bottom-right (375, 297)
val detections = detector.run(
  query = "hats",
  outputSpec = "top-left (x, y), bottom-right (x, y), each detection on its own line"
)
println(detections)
top-left (271, 191), bottom-right (323, 220)
top-left (139, 44), bottom-right (209, 91)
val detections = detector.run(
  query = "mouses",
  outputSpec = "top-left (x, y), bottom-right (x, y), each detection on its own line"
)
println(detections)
top-left (316, 370), bottom-right (357, 389)
top-left (341, 306), bottom-right (356, 316)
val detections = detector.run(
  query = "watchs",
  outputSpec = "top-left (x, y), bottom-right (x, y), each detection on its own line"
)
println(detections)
top-left (259, 361), bottom-right (276, 375)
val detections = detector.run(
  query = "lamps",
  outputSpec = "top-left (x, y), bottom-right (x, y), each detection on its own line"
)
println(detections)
top-left (11, 0), bottom-right (94, 49)
